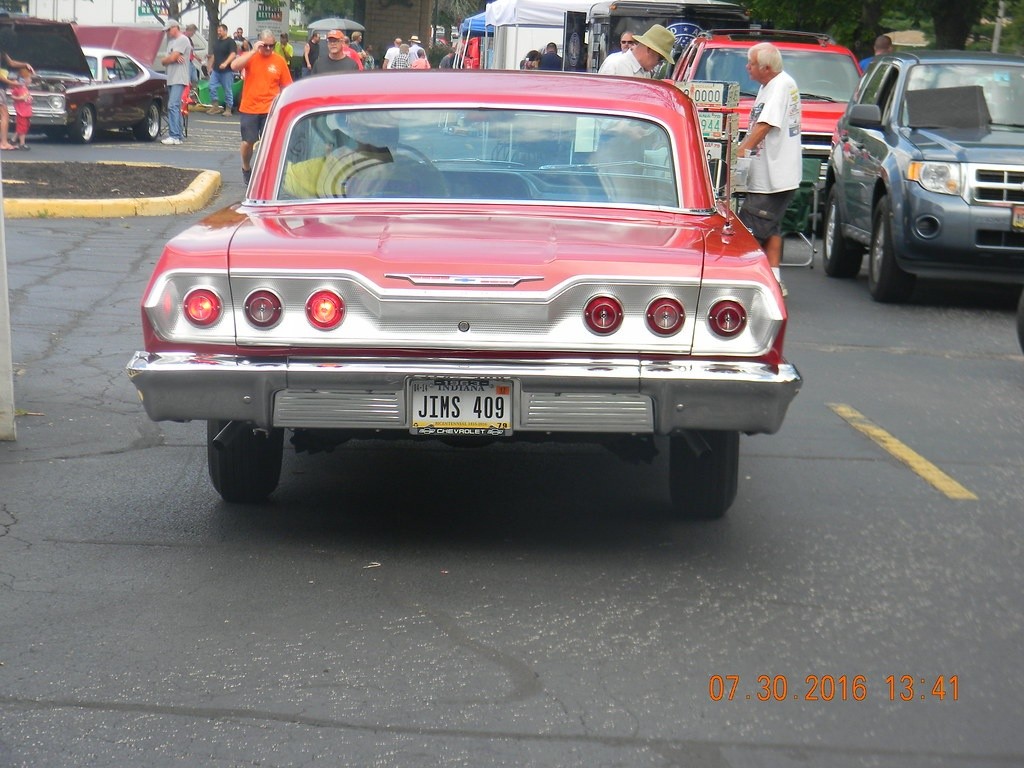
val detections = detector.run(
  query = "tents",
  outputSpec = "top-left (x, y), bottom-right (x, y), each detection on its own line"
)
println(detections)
top-left (452, 0), bottom-right (592, 71)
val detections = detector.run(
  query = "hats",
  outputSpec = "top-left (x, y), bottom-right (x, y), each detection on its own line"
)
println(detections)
top-left (187, 25), bottom-right (198, 32)
top-left (633, 23), bottom-right (675, 65)
top-left (327, 30), bottom-right (344, 40)
top-left (408, 36), bottom-right (421, 43)
top-left (162, 19), bottom-right (179, 32)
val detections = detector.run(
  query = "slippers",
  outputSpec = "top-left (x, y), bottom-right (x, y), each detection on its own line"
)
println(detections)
top-left (0, 144), bottom-right (19, 151)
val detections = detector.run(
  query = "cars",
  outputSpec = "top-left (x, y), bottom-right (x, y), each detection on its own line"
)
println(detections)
top-left (0, 13), bottom-right (170, 143)
top-left (127, 65), bottom-right (805, 517)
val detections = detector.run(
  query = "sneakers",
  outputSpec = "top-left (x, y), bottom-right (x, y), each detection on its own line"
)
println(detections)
top-left (161, 136), bottom-right (183, 145)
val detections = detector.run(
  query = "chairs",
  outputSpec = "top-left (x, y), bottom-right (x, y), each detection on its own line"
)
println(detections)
top-left (778, 158), bottom-right (822, 267)
top-left (159, 83), bottom-right (192, 137)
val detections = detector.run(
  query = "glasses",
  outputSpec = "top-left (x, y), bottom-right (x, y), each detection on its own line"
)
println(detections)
top-left (620, 40), bottom-right (634, 45)
top-left (262, 44), bottom-right (275, 48)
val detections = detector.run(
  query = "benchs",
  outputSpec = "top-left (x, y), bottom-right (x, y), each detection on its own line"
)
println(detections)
top-left (440, 169), bottom-right (606, 202)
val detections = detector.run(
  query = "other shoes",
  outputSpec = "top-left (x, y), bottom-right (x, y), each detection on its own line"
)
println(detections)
top-left (779, 283), bottom-right (787, 297)
top-left (222, 111), bottom-right (232, 117)
top-left (17, 143), bottom-right (31, 151)
top-left (9, 139), bottom-right (20, 147)
top-left (242, 167), bottom-right (251, 185)
top-left (207, 108), bottom-right (220, 114)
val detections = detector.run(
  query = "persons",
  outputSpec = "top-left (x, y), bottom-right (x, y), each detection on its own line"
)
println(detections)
top-left (302, 33), bottom-right (320, 77)
top-left (735, 42), bottom-right (803, 297)
top-left (311, 30), bottom-right (359, 74)
top-left (278, 111), bottom-right (398, 199)
top-left (439, 47), bottom-right (466, 69)
top-left (231, 30), bottom-right (293, 182)
top-left (232, 27), bottom-right (253, 54)
top-left (206, 24), bottom-right (237, 116)
top-left (608, 31), bottom-right (634, 55)
top-left (343, 32), bottom-right (374, 70)
top-left (411, 48), bottom-right (430, 69)
top-left (408, 35), bottom-right (427, 62)
top-left (538, 42), bottom-right (563, 71)
top-left (391, 44), bottom-right (410, 69)
top-left (520, 50), bottom-right (541, 70)
top-left (382, 38), bottom-right (402, 69)
top-left (160, 19), bottom-right (202, 144)
top-left (859, 35), bottom-right (892, 73)
top-left (594, 23), bottom-right (676, 173)
top-left (274, 32), bottom-right (293, 69)
top-left (0, 27), bottom-right (35, 149)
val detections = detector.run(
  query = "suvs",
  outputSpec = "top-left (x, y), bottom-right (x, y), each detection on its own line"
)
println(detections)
top-left (821, 51), bottom-right (1024, 304)
top-left (670, 28), bottom-right (864, 216)
